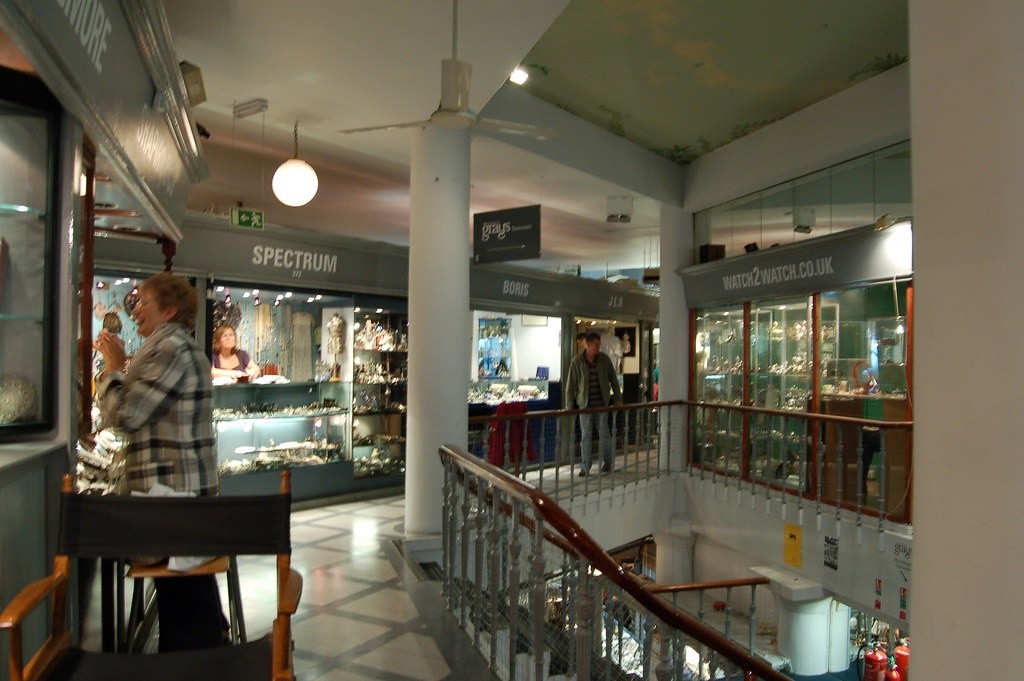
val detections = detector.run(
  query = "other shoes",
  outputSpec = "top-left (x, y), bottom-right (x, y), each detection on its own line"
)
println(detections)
top-left (578, 468), bottom-right (589, 476)
top-left (602, 463), bottom-right (611, 472)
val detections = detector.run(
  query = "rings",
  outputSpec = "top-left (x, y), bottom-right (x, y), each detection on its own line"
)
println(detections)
top-left (97, 341), bottom-right (104, 346)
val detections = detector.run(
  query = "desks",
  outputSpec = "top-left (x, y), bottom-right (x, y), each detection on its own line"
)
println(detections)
top-left (807, 390), bottom-right (913, 526)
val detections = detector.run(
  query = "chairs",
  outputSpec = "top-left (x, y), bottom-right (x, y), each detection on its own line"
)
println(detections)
top-left (0, 468), bottom-right (303, 681)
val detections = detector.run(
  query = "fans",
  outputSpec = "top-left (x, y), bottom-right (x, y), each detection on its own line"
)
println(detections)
top-left (340, 0), bottom-right (567, 146)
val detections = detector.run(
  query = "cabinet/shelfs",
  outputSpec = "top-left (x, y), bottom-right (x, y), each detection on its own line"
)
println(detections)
top-left (322, 292), bottom-right (409, 503)
top-left (212, 380), bottom-right (351, 502)
top-left (0, 65), bottom-right (64, 443)
top-left (689, 295), bottom-right (840, 497)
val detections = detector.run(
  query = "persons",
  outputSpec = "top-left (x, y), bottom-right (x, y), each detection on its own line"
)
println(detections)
top-left (565, 332), bottom-right (624, 477)
top-left (92, 270), bottom-right (236, 661)
top-left (575, 333), bottom-right (589, 352)
top-left (210, 325), bottom-right (262, 384)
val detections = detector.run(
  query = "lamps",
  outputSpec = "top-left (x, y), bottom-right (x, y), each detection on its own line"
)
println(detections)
top-left (271, 120), bottom-right (319, 207)
top-left (872, 214), bottom-right (900, 234)
